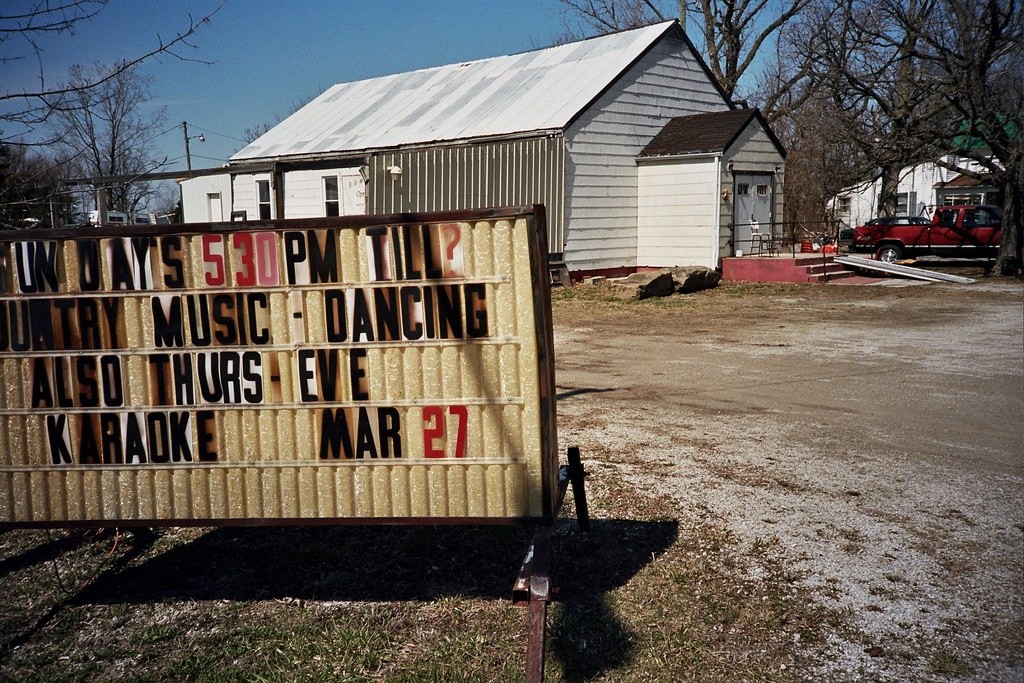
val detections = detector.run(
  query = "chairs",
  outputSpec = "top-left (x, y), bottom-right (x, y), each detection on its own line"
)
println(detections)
top-left (966, 214), bottom-right (977, 225)
top-left (943, 214), bottom-right (954, 224)
top-left (761, 241), bottom-right (779, 258)
top-left (748, 220), bottom-right (770, 258)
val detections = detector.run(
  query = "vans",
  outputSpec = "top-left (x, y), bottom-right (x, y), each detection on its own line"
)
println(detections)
top-left (128, 212), bottom-right (151, 225)
top-left (87, 210), bottom-right (127, 227)
top-left (932, 204), bottom-right (1003, 247)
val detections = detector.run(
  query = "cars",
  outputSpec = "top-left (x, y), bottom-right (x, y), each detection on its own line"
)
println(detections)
top-left (851, 216), bottom-right (975, 265)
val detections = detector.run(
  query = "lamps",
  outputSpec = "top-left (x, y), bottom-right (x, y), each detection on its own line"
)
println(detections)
top-left (387, 165), bottom-right (403, 180)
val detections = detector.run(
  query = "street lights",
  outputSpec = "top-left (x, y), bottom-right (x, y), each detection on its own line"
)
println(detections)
top-left (184, 133), bottom-right (204, 175)
